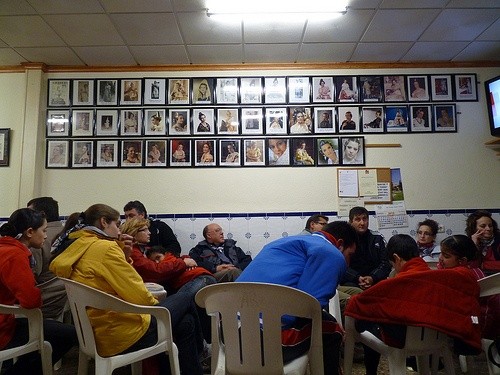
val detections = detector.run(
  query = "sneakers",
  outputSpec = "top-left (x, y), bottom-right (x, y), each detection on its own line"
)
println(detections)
top-left (198, 338), bottom-right (211, 370)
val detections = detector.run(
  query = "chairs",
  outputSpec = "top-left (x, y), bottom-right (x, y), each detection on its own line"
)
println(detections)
top-left (0, 304), bottom-right (52, 375)
top-left (458, 273), bottom-right (500, 375)
top-left (344, 266), bottom-right (481, 375)
top-left (58, 275), bottom-right (180, 375)
top-left (195, 282), bottom-right (324, 375)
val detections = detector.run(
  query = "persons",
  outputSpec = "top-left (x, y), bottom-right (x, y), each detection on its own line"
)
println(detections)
top-left (298, 206), bottom-right (500, 314)
top-left (49, 204), bottom-right (212, 375)
top-left (118, 200), bottom-right (219, 375)
top-left (0, 208), bottom-right (77, 375)
top-left (362, 234), bottom-right (433, 375)
top-left (45, 73), bottom-right (479, 170)
top-left (189, 223), bottom-right (252, 283)
top-left (26, 197), bottom-right (64, 318)
top-left (234, 221), bottom-right (358, 375)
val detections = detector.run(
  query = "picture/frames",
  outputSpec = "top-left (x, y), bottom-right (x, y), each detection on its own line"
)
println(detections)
top-left (45, 73), bottom-right (479, 169)
top-left (0, 127), bottom-right (10, 166)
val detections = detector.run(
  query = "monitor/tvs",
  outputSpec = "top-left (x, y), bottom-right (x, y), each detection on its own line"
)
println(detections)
top-left (484, 75), bottom-right (500, 136)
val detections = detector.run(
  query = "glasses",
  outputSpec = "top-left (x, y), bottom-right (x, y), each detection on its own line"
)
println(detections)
top-left (316, 223), bottom-right (327, 225)
top-left (137, 228), bottom-right (149, 233)
top-left (417, 231), bottom-right (433, 236)
